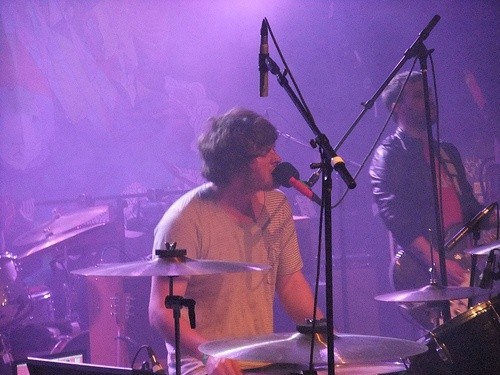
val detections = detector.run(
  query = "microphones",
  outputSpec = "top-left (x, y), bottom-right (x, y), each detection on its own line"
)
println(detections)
top-left (443, 202), bottom-right (496, 251)
top-left (259, 24), bottom-right (268, 97)
top-left (272, 162), bottom-right (324, 207)
top-left (147, 348), bottom-right (165, 375)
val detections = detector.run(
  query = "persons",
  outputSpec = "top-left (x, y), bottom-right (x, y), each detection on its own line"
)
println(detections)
top-left (370, 70), bottom-right (500, 330)
top-left (149, 107), bottom-right (338, 375)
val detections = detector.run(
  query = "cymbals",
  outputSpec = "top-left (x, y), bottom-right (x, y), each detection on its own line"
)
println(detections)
top-left (372, 282), bottom-right (498, 304)
top-left (16, 221), bottom-right (106, 262)
top-left (66, 256), bottom-right (276, 278)
top-left (462, 240), bottom-right (500, 255)
top-left (293, 214), bottom-right (310, 222)
top-left (9, 202), bottom-right (110, 247)
top-left (75, 227), bottom-right (144, 246)
top-left (197, 329), bottom-right (430, 365)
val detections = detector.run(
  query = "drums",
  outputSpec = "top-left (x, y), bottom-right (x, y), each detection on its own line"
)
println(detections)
top-left (399, 298), bottom-right (500, 375)
top-left (11, 319), bottom-right (90, 363)
top-left (312, 361), bottom-right (409, 375)
top-left (0, 251), bottom-right (34, 331)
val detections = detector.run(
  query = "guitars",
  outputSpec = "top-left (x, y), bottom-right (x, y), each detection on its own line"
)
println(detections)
top-left (391, 220), bottom-right (500, 334)
top-left (105, 290), bottom-right (137, 368)
